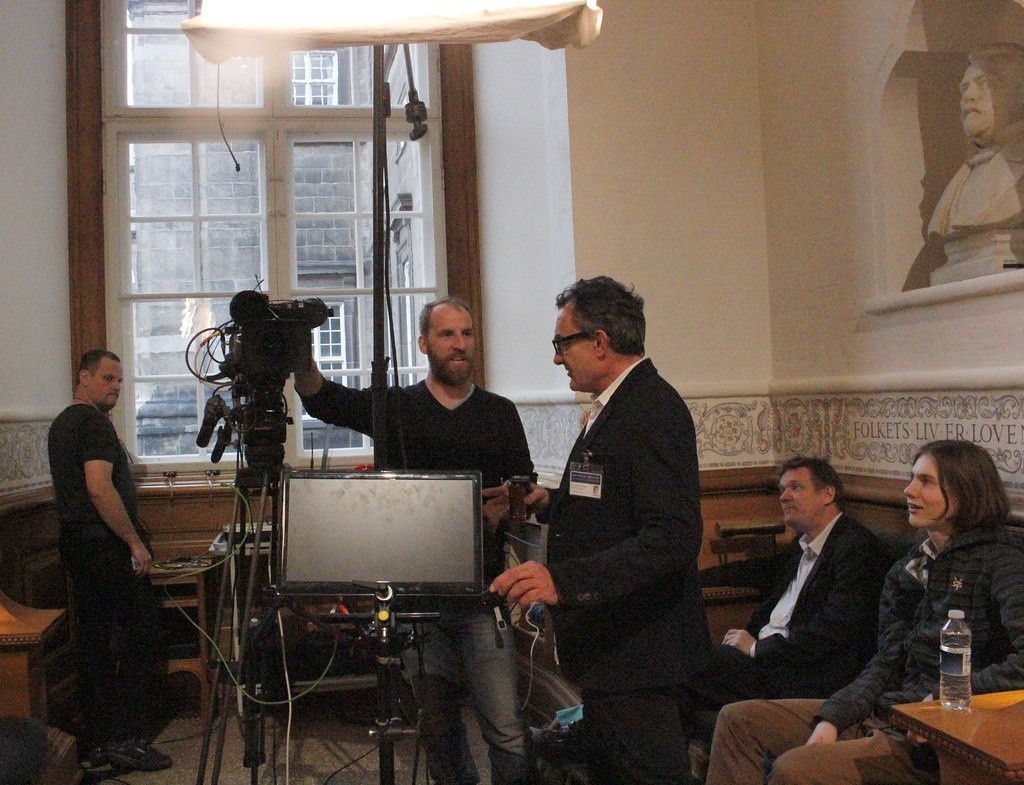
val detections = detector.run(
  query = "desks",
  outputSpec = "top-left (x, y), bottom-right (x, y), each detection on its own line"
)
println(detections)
top-left (149, 570), bottom-right (211, 720)
top-left (208, 532), bottom-right (379, 726)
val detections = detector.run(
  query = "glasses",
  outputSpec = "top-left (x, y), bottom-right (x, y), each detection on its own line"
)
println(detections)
top-left (552, 332), bottom-right (589, 354)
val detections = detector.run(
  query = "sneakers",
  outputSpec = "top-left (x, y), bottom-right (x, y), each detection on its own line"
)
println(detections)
top-left (89, 737), bottom-right (173, 774)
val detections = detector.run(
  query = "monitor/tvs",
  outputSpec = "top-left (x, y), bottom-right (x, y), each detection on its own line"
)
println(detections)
top-left (277, 470), bottom-right (484, 596)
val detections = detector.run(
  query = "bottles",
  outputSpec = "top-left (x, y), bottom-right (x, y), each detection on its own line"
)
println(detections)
top-left (940, 609), bottom-right (972, 708)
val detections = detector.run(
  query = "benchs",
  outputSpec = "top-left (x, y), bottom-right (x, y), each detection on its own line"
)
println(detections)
top-left (688, 585), bottom-right (1024, 785)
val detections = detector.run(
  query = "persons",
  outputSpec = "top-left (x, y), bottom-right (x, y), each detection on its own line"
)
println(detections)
top-left (928, 42), bottom-right (1024, 286)
top-left (48, 349), bottom-right (172, 771)
top-left (294, 297), bottom-right (539, 785)
top-left (593, 486), bottom-right (599, 494)
top-left (482, 276), bottom-right (705, 785)
top-left (694, 440), bottom-right (1023, 785)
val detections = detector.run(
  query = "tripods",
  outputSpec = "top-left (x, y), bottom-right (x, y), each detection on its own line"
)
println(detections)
top-left (195, 400), bottom-right (294, 785)
